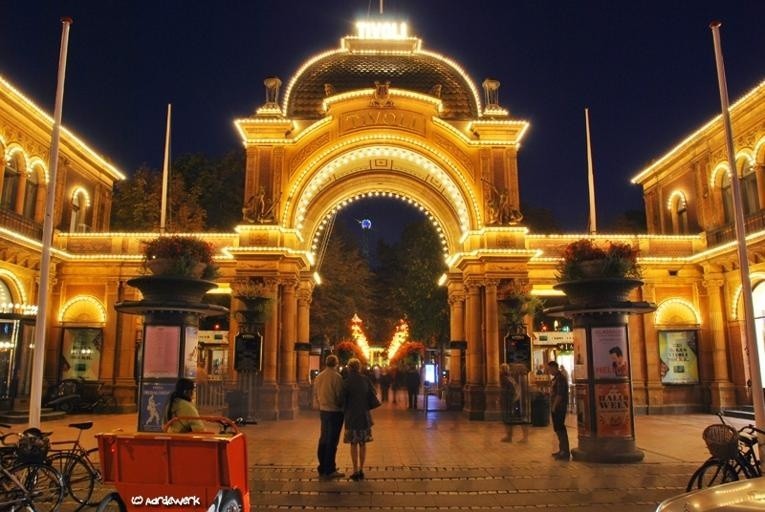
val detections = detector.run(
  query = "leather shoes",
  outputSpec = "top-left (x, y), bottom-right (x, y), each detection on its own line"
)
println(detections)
top-left (350, 470), bottom-right (366, 482)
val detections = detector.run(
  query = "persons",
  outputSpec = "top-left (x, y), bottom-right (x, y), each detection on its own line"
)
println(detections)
top-left (161, 377), bottom-right (206, 434)
top-left (371, 360), bottom-right (421, 409)
top-left (546, 361), bottom-right (570, 461)
top-left (500, 363), bottom-right (528, 443)
top-left (536, 364), bottom-right (545, 374)
top-left (312, 353), bottom-right (345, 479)
top-left (333, 357), bottom-right (377, 482)
top-left (609, 346), bottom-right (629, 376)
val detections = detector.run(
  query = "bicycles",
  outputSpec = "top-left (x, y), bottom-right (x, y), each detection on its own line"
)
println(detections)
top-left (0, 420), bottom-right (117, 511)
top-left (688, 408), bottom-right (765, 492)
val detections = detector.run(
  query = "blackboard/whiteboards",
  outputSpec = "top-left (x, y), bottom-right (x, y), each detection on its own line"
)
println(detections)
top-left (233, 331), bottom-right (263, 372)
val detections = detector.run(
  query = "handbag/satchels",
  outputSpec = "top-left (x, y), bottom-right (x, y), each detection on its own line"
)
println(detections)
top-left (365, 383), bottom-right (382, 411)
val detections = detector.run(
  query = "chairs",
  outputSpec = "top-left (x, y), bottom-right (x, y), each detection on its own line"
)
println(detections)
top-left (45, 378), bottom-right (120, 415)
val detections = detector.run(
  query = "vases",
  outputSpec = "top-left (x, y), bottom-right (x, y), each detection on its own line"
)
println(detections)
top-left (144, 257), bottom-right (206, 279)
top-left (235, 295), bottom-right (273, 311)
top-left (497, 297), bottom-right (533, 313)
top-left (568, 256), bottom-right (635, 280)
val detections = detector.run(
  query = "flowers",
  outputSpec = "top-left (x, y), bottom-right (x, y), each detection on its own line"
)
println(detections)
top-left (551, 237), bottom-right (647, 284)
top-left (498, 281), bottom-right (540, 299)
top-left (234, 282), bottom-right (277, 299)
top-left (134, 232), bottom-right (224, 284)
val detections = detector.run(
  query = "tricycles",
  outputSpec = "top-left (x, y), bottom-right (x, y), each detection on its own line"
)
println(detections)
top-left (79, 411), bottom-right (254, 512)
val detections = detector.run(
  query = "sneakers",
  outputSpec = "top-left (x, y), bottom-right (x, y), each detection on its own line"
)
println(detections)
top-left (551, 450), bottom-right (571, 460)
top-left (318, 467), bottom-right (346, 478)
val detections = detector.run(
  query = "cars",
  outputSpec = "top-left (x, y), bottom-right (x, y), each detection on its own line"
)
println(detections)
top-left (654, 475), bottom-right (765, 512)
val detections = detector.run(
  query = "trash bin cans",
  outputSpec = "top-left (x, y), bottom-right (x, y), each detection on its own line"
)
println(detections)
top-left (531, 396), bottom-right (549, 427)
top-left (227, 390), bottom-right (247, 424)
